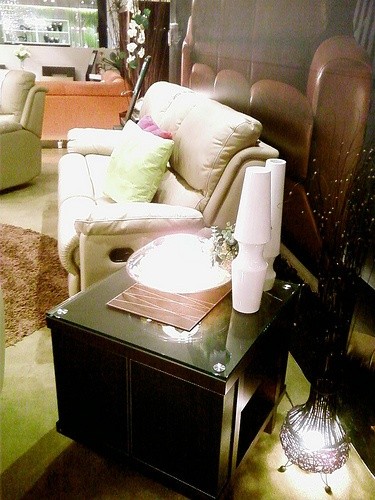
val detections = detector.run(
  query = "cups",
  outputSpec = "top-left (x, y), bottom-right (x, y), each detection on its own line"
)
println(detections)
top-left (46, 21), bottom-right (62, 32)
top-left (44, 34), bottom-right (60, 43)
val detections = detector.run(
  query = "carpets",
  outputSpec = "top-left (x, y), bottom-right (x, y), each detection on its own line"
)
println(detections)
top-left (0, 225), bottom-right (68, 348)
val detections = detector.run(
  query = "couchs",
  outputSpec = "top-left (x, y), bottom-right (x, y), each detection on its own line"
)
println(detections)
top-left (56, 80), bottom-right (280, 302)
top-left (0, 69), bottom-right (50, 193)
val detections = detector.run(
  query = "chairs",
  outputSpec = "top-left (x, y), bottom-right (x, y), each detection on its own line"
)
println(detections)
top-left (187, 35), bottom-right (373, 267)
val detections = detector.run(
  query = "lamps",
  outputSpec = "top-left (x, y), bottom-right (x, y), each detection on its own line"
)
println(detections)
top-left (125, 234), bottom-right (233, 293)
top-left (277, 138), bottom-right (375, 493)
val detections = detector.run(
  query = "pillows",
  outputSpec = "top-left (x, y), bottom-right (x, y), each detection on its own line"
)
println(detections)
top-left (101, 112), bottom-right (175, 204)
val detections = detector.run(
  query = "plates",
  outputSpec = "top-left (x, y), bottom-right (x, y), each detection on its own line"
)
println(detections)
top-left (125, 231), bottom-right (231, 294)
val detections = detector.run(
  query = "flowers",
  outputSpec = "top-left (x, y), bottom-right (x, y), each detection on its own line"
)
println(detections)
top-left (124, 0), bottom-right (151, 71)
top-left (200, 221), bottom-right (239, 273)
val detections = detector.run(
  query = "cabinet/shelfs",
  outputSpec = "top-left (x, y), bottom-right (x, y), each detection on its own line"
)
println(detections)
top-left (2, 17), bottom-right (71, 46)
top-left (44, 265), bottom-right (300, 500)
